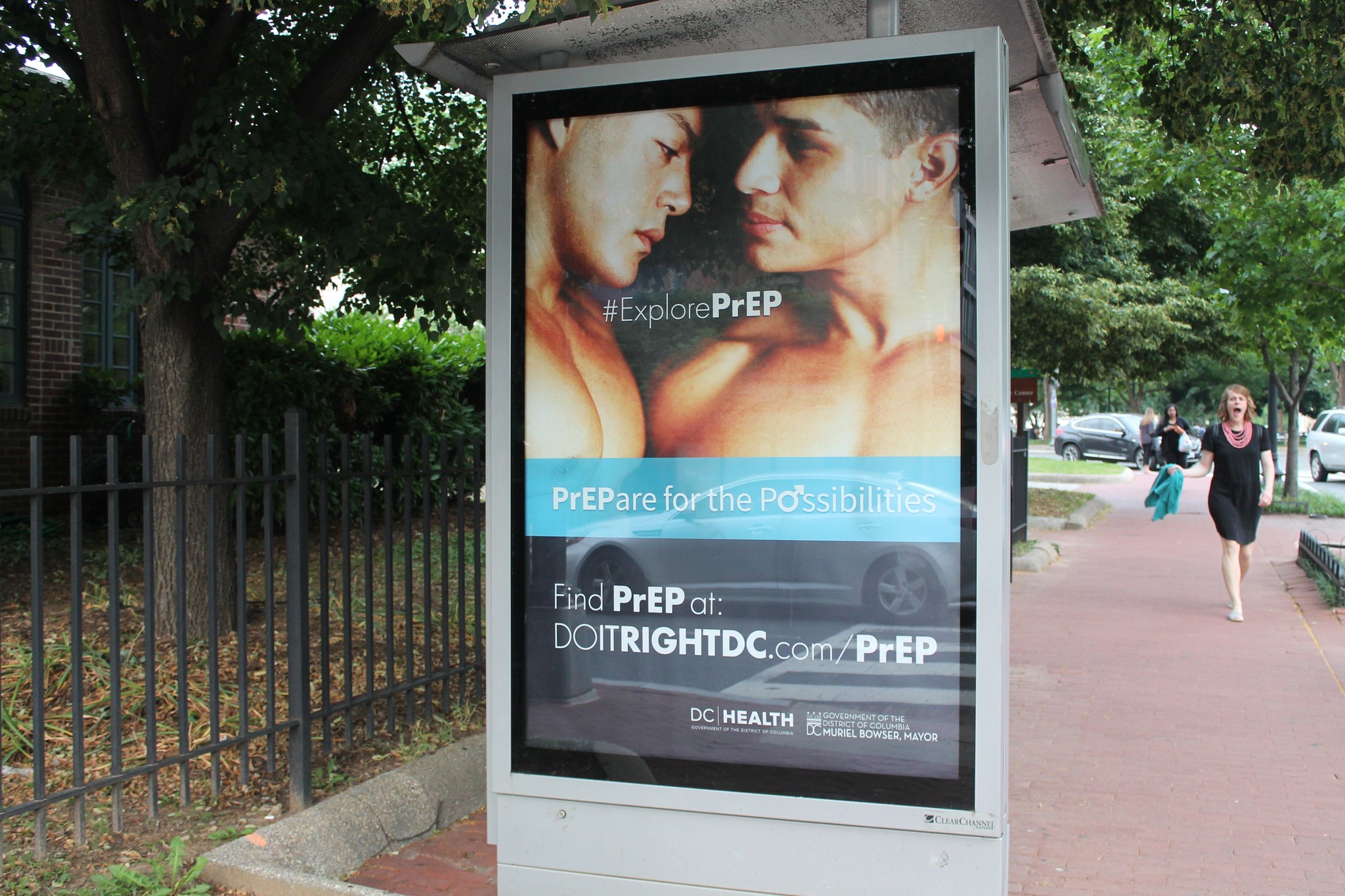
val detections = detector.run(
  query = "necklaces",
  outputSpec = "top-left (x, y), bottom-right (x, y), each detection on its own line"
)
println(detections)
top-left (1222, 420), bottom-right (1252, 448)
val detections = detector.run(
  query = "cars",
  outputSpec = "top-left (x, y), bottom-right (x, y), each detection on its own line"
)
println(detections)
top-left (1054, 412), bottom-right (1203, 471)
top-left (1304, 406), bottom-right (1345, 482)
top-left (563, 472), bottom-right (977, 623)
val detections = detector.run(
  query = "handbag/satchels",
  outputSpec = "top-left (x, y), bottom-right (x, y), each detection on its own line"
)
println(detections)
top-left (1178, 431), bottom-right (1193, 452)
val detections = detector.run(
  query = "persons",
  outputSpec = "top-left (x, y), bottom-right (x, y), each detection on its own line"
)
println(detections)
top-left (1139, 408), bottom-right (1157, 471)
top-left (525, 78), bottom-right (963, 459)
top-left (1167, 384), bottom-right (1274, 622)
top-left (1153, 404), bottom-right (1192, 469)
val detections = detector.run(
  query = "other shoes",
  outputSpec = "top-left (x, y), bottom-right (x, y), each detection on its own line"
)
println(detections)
top-left (1228, 601), bottom-right (1233, 608)
top-left (1228, 612), bottom-right (1244, 622)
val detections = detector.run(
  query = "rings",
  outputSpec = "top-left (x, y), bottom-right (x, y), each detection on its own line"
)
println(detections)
top-left (1265, 499), bottom-right (1268, 502)
